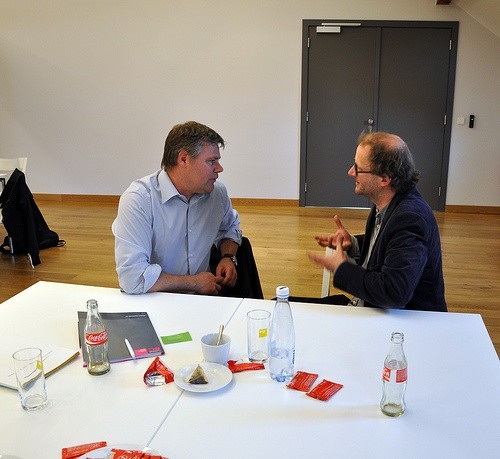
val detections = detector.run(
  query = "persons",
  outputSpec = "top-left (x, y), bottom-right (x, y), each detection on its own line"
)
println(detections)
top-left (112, 120), bottom-right (242, 298)
top-left (307, 131), bottom-right (448, 312)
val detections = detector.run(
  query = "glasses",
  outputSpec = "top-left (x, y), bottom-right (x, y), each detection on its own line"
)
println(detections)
top-left (353, 162), bottom-right (371, 176)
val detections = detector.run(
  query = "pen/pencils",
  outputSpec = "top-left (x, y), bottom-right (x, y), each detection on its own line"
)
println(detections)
top-left (125, 339), bottom-right (135, 358)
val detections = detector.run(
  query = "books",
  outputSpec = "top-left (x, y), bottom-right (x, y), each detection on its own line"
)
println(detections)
top-left (77, 311), bottom-right (164, 368)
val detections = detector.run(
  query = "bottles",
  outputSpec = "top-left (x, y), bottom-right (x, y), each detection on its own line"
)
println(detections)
top-left (84, 298), bottom-right (111, 377)
top-left (380, 332), bottom-right (408, 417)
top-left (269, 286), bottom-right (295, 383)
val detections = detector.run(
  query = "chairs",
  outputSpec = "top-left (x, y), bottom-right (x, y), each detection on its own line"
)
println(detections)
top-left (0, 169), bottom-right (35, 271)
top-left (209, 237), bottom-right (265, 299)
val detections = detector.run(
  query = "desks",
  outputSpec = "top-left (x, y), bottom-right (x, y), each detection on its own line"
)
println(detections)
top-left (0, 281), bottom-right (500, 459)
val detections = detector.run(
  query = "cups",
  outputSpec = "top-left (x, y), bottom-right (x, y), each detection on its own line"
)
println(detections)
top-left (247, 309), bottom-right (271, 363)
top-left (12, 347), bottom-right (50, 412)
top-left (202, 332), bottom-right (231, 364)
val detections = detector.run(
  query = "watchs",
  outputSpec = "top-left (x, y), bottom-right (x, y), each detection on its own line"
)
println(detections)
top-left (221, 254), bottom-right (238, 266)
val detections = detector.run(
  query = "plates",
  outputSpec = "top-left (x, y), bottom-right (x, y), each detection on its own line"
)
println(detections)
top-left (174, 361), bottom-right (233, 393)
